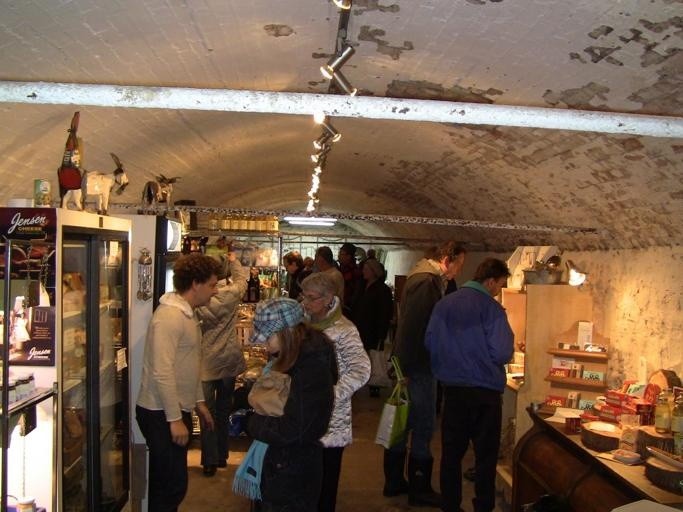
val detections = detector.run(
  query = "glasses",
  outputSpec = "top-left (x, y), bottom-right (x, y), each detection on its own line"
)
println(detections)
top-left (300, 293), bottom-right (322, 301)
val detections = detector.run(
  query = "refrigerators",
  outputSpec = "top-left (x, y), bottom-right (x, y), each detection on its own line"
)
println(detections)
top-left (106, 210), bottom-right (182, 510)
top-left (0, 206), bottom-right (133, 511)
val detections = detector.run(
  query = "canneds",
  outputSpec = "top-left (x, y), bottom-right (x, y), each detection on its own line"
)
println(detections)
top-left (0, 373), bottom-right (35, 408)
top-left (34, 178), bottom-right (53, 208)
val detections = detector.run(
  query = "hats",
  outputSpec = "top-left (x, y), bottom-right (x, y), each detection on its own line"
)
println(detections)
top-left (248, 298), bottom-right (302, 344)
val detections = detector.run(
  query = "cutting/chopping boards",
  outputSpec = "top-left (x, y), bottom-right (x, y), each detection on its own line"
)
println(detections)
top-left (579, 414), bottom-right (683, 497)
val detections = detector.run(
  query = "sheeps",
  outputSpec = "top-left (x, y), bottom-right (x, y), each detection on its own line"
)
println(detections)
top-left (141, 174), bottom-right (183, 215)
top-left (58, 152), bottom-right (128, 215)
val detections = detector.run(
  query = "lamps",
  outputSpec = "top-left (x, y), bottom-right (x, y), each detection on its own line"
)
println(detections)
top-left (563, 258), bottom-right (589, 288)
top-left (136, 243), bottom-right (153, 301)
top-left (308, 0), bottom-right (364, 173)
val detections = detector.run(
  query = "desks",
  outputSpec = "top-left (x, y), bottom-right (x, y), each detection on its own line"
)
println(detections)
top-left (505, 399), bottom-right (682, 512)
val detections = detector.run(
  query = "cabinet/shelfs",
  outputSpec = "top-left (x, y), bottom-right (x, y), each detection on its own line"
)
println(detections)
top-left (182, 203), bottom-right (284, 437)
top-left (492, 282), bottom-right (593, 499)
top-left (537, 347), bottom-right (608, 414)
top-left (0, 239), bottom-right (60, 512)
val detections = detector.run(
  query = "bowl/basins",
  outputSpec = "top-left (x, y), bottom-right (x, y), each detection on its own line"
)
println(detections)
top-left (521, 268), bottom-right (563, 285)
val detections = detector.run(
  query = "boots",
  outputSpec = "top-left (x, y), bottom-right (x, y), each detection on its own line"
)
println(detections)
top-left (383, 449), bottom-right (441, 507)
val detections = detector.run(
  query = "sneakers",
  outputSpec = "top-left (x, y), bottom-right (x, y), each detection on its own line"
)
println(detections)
top-left (203, 460), bottom-right (226, 476)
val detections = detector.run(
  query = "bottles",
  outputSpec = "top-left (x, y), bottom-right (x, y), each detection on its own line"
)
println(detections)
top-left (653, 386), bottom-right (683, 434)
top-left (207, 210), bottom-right (279, 234)
top-left (242, 277), bottom-right (262, 302)
top-left (15, 497), bottom-right (35, 512)
top-left (0, 370), bottom-right (34, 409)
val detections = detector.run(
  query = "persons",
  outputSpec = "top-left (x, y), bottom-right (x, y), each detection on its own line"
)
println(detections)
top-left (300, 269), bottom-right (371, 511)
top-left (423, 257), bottom-right (513, 510)
top-left (133, 252), bottom-right (223, 511)
top-left (198, 248), bottom-right (247, 478)
top-left (382, 240), bottom-right (467, 510)
top-left (231, 297), bottom-right (332, 511)
top-left (283, 243), bottom-right (394, 353)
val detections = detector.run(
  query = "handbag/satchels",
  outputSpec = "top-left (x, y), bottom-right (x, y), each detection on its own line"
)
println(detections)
top-left (367, 349), bottom-right (394, 387)
top-left (375, 398), bottom-right (409, 449)
top-left (248, 371), bottom-right (291, 417)
